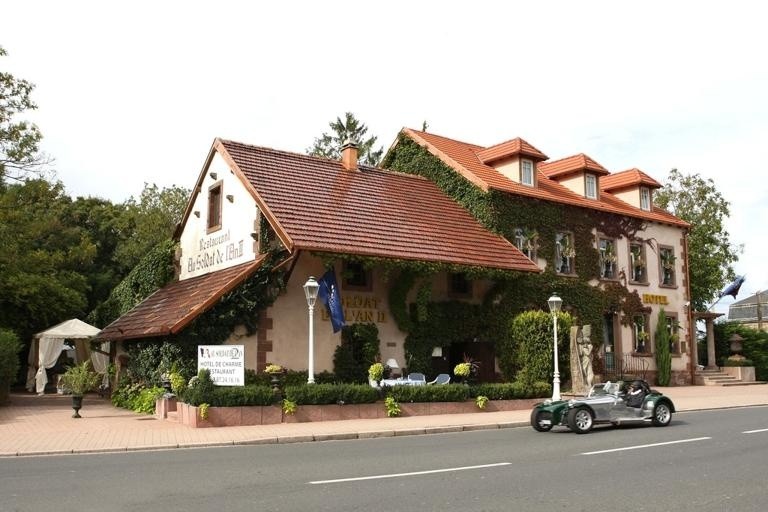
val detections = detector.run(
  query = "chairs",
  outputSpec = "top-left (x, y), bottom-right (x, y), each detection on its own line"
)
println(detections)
top-left (408, 372), bottom-right (450, 386)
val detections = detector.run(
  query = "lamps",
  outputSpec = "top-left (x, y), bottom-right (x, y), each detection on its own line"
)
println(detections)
top-left (386, 359), bottom-right (399, 377)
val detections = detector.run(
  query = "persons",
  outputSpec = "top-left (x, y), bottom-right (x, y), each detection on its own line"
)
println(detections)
top-left (625, 380), bottom-right (645, 408)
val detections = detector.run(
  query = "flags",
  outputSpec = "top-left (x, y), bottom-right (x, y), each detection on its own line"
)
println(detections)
top-left (316, 263), bottom-right (346, 334)
top-left (722, 275), bottom-right (745, 299)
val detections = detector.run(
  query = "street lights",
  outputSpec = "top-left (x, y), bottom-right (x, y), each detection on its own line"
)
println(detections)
top-left (302, 276), bottom-right (320, 384)
top-left (547, 292), bottom-right (564, 401)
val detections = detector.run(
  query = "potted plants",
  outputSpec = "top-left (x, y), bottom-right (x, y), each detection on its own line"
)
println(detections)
top-left (264, 364), bottom-right (286, 391)
top-left (60, 360), bottom-right (106, 419)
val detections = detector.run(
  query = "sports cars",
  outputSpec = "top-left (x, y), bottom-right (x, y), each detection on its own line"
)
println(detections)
top-left (531, 378), bottom-right (676, 435)
